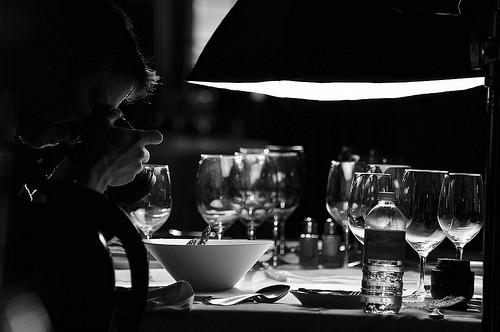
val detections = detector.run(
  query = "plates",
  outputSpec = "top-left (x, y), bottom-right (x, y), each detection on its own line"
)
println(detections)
top-left (291, 289), bottom-right (362, 308)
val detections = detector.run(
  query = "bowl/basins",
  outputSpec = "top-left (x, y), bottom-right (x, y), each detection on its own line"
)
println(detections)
top-left (142, 237), bottom-right (275, 291)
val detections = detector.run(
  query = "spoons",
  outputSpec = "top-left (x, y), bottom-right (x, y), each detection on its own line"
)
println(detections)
top-left (205, 284), bottom-right (290, 305)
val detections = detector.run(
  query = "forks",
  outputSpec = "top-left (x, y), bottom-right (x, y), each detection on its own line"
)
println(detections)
top-left (298, 288), bottom-right (361, 296)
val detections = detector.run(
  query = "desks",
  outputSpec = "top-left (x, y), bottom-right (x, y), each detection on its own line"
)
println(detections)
top-left (115, 242), bottom-right (484, 332)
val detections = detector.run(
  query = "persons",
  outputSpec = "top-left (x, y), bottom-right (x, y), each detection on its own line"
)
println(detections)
top-left (0, 0), bottom-right (164, 200)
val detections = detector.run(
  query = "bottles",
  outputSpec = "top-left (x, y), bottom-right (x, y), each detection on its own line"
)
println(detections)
top-left (321, 221), bottom-right (340, 268)
top-left (360, 190), bottom-right (407, 316)
top-left (300, 220), bottom-right (319, 271)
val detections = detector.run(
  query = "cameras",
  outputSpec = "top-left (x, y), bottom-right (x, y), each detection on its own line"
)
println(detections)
top-left (30, 118), bottom-right (157, 207)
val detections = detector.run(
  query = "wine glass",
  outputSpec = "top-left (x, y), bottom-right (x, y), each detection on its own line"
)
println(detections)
top-left (198, 144), bottom-right (304, 274)
top-left (325, 159), bottom-right (484, 307)
top-left (126, 164), bottom-right (173, 286)
